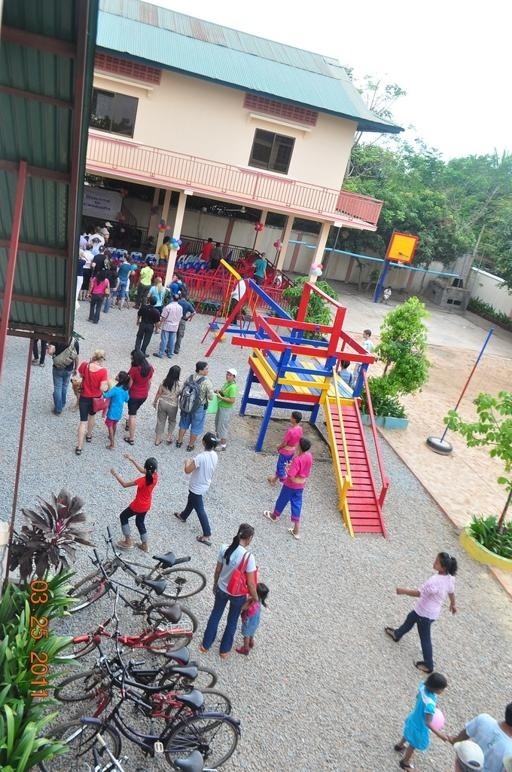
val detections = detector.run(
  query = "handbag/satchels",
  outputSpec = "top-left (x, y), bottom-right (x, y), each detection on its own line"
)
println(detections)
top-left (207, 393), bottom-right (222, 414)
top-left (53, 342), bottom-right (77, 369)
top-left (92, 396), bottom-right (111, 414)
top-left (71, 374), bottom-right (82, 397)
top-left (227, 566), bottom-right (258, 595)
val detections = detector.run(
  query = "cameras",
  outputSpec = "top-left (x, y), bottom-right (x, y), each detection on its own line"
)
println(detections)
top-left (218, 391), bottom-right (224, 397)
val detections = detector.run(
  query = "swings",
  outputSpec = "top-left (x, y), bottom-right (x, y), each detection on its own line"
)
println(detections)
top-left (209, 269), bottom-right (236, 340)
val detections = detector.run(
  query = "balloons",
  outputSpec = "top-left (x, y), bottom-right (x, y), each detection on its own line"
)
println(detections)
top-left (310, 262), bottom-right (324, 278)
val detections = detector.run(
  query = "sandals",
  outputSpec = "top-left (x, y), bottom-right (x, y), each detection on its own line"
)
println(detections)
top-left (76, 419), bottom-right (134, 455)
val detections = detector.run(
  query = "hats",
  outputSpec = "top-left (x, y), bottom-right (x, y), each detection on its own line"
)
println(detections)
top-left (226, 368), bottom-right (238, 378)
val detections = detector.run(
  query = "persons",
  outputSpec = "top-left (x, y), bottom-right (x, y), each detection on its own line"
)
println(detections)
top-left (135, 273), bottom-right (196, 359)
top-left (273, 269), bottom-right (282, 289)
top-left (201, 239), bottom-right (222, 270)
top-left (234, 581), bottom-right (270, 656)
top-left (173, 431), bottom-right (219, 546)
top-left (253, 252), bottom-right (267, 286)
top-left (392, 672), bottom-right (449, 772)
top-left (446, 702), bottom-right (511, 772)
top-left (228, 275), bottom-right (246, 325)
top-left (267, 410), bottom-right (304, 488)
top-left (75, 221), bottom-right (154, 325)
top-left (34, 330), bottom-right (237, 455)
top-left (263, 437), bottom-right (313, 539)
top-left (198, 522), bottom-right (260, 657)
top-left (383, 551), bottom-right (458, 674)
top-left (157, 237), bottom-right (170, 266)
top-left (353, 328), bottom-right (374, 377)
top-left (323, 359), bottom-right (354, 427)
top-left (111, 452), bottom-right (158, 552)
top-left (364, 269), bottom-right (393, 305)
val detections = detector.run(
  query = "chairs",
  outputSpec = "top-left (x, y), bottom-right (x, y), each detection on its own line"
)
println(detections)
top-left (109, 245), bottom-right (290, 304)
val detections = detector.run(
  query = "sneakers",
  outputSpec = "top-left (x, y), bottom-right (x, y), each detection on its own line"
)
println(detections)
top-left (236, 646), bottom-right (250, 655)
top-left (215, 443), bottom-right (226, 451)
top-left (414, 661), bottom-right (433, 672)
top-left (399, 759), bottom-right (414, 769)
top-left (395, 743), bottom-right (407, 750)
top-left (385, 626), bottom-right (400, 642)
top-left (155, 435), bottom-right (194, 452)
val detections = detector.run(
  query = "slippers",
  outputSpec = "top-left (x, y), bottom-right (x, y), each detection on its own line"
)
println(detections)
top-left (288, 527), bottom-right (302, 540)
top-left (136, 542), bottom-right (149, 554)
top-left (264, 510), bottom-right (279, 522)
top-left (219, 653), bottom-right (229, 660)
top-left (197, 535), bottom-right (213, 546)
top-left (199, 645), bottom-right (206, 653)
top-left (174, 511), bottom-right (186, 523)
top-left (116, 540), bottom-right (135, 549)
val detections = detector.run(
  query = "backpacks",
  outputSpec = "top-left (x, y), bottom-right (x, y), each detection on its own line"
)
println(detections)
top-left (179, 375), bottom-right (206, 414)
